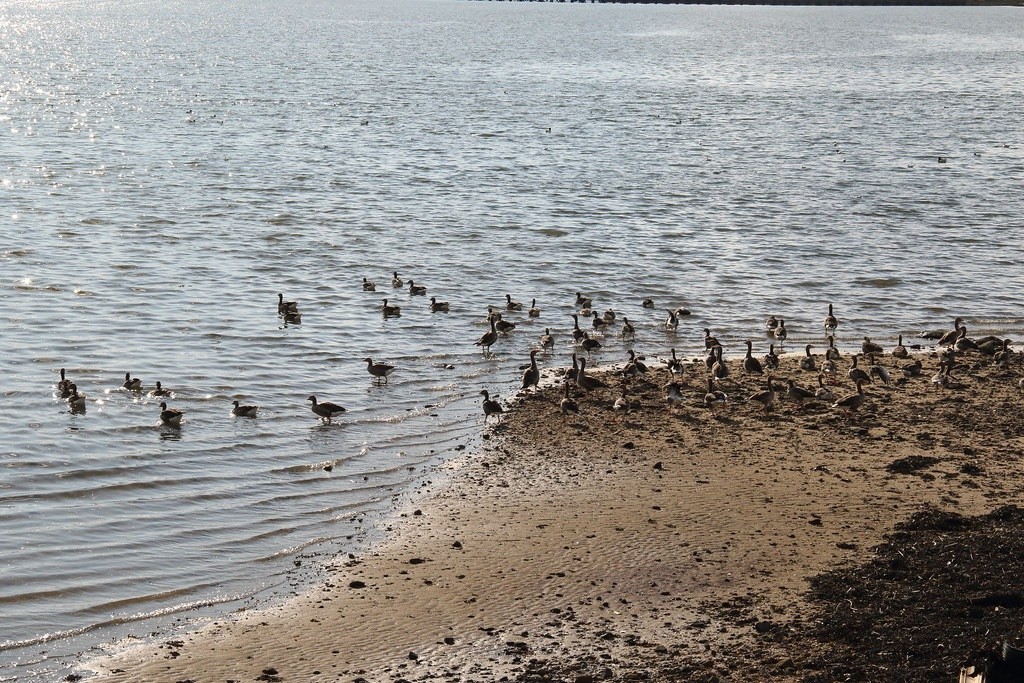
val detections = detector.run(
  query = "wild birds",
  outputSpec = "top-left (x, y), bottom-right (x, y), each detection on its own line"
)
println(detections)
top-left (528, 299), bottom-right (541, 318)
top-left (406, 280), bottom-right (427, 294)
top-left (278, 293), bottom-right (302, 321)
top-left (540, 328), bottom-right (554, 354)
top-left (938, 156), bottom-right (946, 163)
top-left (480, 390), bottom-right (504, 422)
top-left (364, 358), bottom-right (396, 383)
top-left (561, 290), bottom-right (1013, 418)
top-left (58, 368), bottom-right (86, 405)
top-left (151, 380), bottom-right (172, 396)
top-left (308, 395), bottom-right (347, 424)
top-left (122, 373), bottom-right (142, 389)
top-left (362, 278), bottom-right (376, 291)
top-left (392, 271), bottom-right (403, 288)
top-left (429, 297), bottom-right (449, 310)
top-left (232, 401), bottom-right (258, 418)
top-left (158, 402), bottom-right (183, 424)
top-left (380, 299), bottom-right (401, 315)
top-left (473, 295), bottom-right (523, 350)
top-left (520, 350), bottom-right (542, 393)
